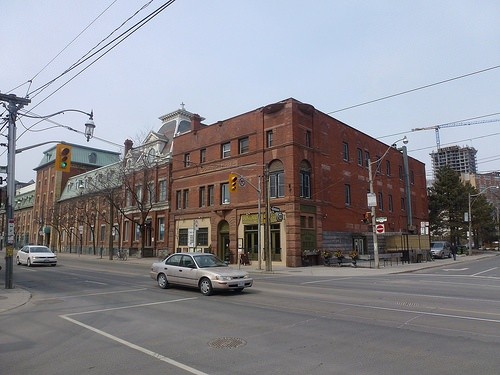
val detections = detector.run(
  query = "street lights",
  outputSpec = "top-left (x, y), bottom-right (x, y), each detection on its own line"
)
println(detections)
top-left (469, 185), bottom-right (499, 256)
top-left (5, 109), bottom-right (96, 289)
top-left (368, 136), bottom-right (409, 269)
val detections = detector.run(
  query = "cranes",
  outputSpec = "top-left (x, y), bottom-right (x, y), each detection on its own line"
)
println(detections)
top-left (415, 118), bottom-right (500, 151)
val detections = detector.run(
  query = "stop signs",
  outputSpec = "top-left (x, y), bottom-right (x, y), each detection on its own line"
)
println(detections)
top-left (376, 223), bottom-right (385, 234)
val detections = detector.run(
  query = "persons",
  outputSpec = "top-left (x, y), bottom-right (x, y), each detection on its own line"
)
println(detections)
top-left (451, 242), bottom-right (458, 261)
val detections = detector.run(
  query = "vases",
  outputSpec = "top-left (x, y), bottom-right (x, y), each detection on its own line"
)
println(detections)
top-left (324, 257), bottom-right (330, 267)
top-left (337, 257), bottom-right (343, 267)
top-left (351, 258), bottom-right (357, 268)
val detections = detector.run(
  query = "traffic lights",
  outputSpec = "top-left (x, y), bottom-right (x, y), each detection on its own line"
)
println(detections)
top-left (229, 173), bottom-right (238, 193)
top-left (55, 144), bottom-right (72, 173)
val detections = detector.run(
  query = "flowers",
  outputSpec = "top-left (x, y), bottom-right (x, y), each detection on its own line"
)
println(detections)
top-left (320, 249), bottom-right (332, 258)
top-left (349, 251), bottom-right (358, 259)
top-left (334, 250), bottom-right (345, 258)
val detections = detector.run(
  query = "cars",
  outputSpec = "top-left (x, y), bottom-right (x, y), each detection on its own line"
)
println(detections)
top-left (151, 252), bottom-right (253, 296)
top-left (16, 244), bottom-right (58, 267)
top-left (429, 241), bottom-right (452, 259)
